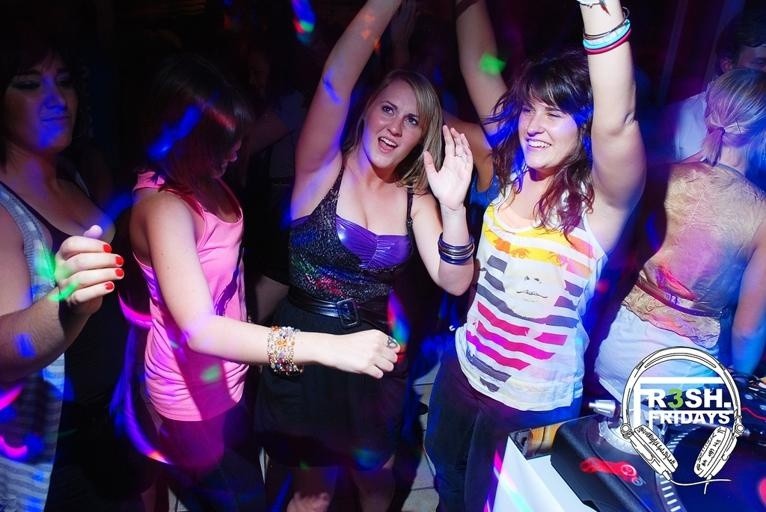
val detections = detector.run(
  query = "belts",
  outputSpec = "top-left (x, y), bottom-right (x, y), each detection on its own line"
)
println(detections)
top-left (289, 290), bottom-right (391, 338)
top-left (634, 273), bottom-right (727, 320)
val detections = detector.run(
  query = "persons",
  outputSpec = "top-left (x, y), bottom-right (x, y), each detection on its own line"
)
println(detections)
top-left (0, 0), bottom-right (766, 512)
